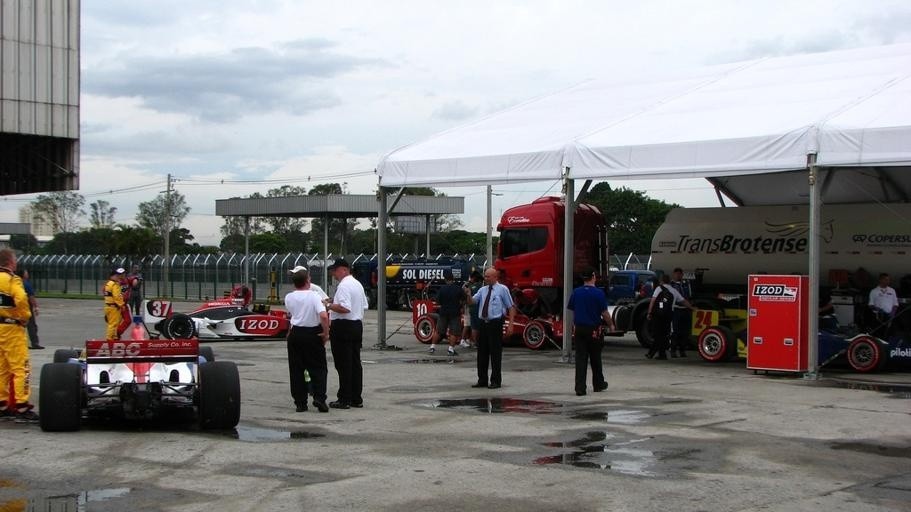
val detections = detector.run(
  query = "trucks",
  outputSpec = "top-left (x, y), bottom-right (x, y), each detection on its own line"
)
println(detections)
top-left (606, 270), bottom-right (657, 305)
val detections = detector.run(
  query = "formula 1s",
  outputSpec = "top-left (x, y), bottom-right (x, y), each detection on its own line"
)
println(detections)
top-left (39, 321), bottom-right (241, 432)
top-left (413, 287), bottom-right (607, 350)
top-left (692, 309), bottom-right (911, 372)
top-left (142, 298), bottom-right (332, 341)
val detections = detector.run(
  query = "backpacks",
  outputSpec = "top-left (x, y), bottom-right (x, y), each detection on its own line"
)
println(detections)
top-left (649, 285), bottom-right (673, 324)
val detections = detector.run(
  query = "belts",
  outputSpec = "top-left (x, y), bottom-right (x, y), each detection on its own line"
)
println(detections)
top-left (484, 320), bottom-right (492, 323)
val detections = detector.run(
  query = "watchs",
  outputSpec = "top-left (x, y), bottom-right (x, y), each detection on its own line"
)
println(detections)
top-left (326, 302), bottom-right (331, 309)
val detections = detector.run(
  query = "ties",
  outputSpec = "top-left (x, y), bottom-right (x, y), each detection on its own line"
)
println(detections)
top-left (481, 287), bottom-right (491, 318)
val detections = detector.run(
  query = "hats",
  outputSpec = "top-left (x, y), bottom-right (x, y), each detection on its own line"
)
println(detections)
top-left (327, 258), bottom-right (347, 270)
top-left (582, 267), bottom-right (597, 276)
top-left (288, 265), bottom-right (306, 274)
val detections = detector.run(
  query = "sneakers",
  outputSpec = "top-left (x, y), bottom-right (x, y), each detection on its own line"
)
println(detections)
top-left (13, 410), bottom-right (39, 424)
top-left (0, 408), bottom-right (15, 422)
top-left (295, 399), bottom-right (362, 412)
top-left (429, 339), bottom-right (477, 357)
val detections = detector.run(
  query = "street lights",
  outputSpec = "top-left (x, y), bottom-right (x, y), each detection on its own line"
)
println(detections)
top-left (163, 214), bottom-right (185, 301)
top-left (487, 192), bottom-right (504, 270)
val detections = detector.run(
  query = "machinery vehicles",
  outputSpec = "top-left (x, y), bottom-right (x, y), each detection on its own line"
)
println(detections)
top-left (495, 197), bottom-right (911, 349)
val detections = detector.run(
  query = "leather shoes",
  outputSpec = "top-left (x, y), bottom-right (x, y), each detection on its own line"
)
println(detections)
top-left (487, 383), bottom-right (500, 389)
top-left (594, 382), bottom-right (607, 392)
top-left (471, 384), bottom-right (487, 387)
top-left (31, 343), bottom-right (46, 349)
top-left (645, 353), bottom-right (686, 359)
top-left (576, 390), bottom-right (586, 395)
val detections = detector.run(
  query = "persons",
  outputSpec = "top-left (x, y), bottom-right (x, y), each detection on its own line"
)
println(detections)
top-left (0, 247), bottom-right (40, 423)
top-left (117, 268), bottom-right (139, 339)
top-left (638, 268), bottom-right (693, 361)
top-left (567, 266), bottom-right (615, 396)
top-left (127, 264), bottom-right (143, 316)
top-left (284, 270), bottom-right (329, 412)
top-left (819, 288), bottom-right (834, 315)
top-left (289, 266), bottom-right (330, 396)
top-left (464, 268), bottom-right (515, 389)
top-left (867, 273), bottom-right (899, 341)
top-left (104, 268), bottom-right (126, 342)
top-left (429, 272), bottom-right (541, 356)
top-left (231, 286), bottom-right (251, 311)
top-left (16, 268), bottom-right (44, 349)
top-left (322, 258), bottom-right (369, 410)
